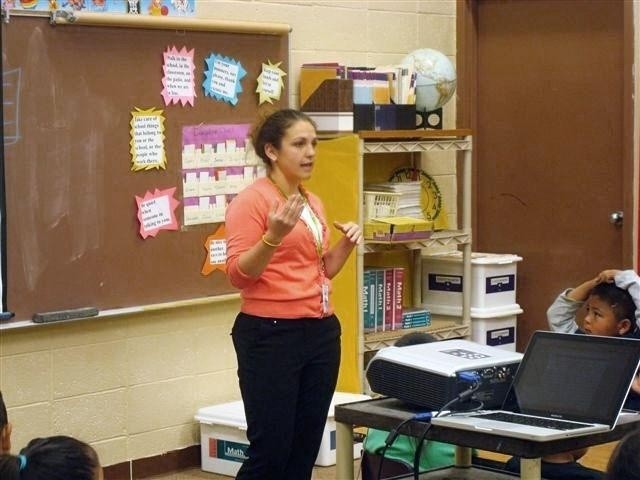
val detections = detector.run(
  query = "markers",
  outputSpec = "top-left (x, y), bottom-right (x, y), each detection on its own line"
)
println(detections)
top-left (1, 312), bottom-right (14, 321)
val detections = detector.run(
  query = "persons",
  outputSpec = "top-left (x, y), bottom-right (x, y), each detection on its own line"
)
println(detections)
top-left (363, 334), bottom-right (479, 472)
top-left (221, 109), bottom-right (363, 479)
top-left (544, 268), bottom-right (640, 338)
top-left (0, 391), bottom-right (12, 454)
top-left (0, 435), bottom-right (105, 480)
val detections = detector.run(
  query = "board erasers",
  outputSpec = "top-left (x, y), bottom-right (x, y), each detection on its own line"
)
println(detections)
top-left (33, 307), bottom-right (98, 323)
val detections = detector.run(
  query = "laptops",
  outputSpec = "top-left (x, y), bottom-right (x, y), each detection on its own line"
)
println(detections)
top-left (429, 329), bottom-right (640, 445)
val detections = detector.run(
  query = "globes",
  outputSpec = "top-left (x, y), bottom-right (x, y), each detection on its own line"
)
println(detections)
top-left (400, 47), bottom-right (457, 129)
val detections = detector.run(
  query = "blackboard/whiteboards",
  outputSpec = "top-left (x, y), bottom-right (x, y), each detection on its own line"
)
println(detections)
top-left (0, 8), bottom-right (293, 330)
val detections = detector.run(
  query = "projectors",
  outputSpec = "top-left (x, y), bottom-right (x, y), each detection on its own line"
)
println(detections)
top-left (364, 339), bottom-right (526, 412)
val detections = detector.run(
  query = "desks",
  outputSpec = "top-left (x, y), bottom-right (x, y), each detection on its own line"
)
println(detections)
top-left (332, 389), bottom-right (639, 480)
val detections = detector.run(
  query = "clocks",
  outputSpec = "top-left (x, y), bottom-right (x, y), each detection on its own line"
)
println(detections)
top-left (386, 165), bottom-right (449, 232)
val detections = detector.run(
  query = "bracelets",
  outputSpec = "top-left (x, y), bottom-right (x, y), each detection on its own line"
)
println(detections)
top-left (262, 234), bottom-right (282, 249)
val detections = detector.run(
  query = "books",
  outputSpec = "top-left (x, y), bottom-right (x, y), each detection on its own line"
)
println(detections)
top-left (299, 61), bottom-right (418, 106)
top-left (362, 176), bottom-right (434, 332)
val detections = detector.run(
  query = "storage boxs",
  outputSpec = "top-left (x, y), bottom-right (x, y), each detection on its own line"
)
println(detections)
top-left (193, 399), bottom-right (268, 479)
top-left (315, 391), bottom-right (373, 469)
top-left (425, 305), bottom-right (524, 354)
top-left (422, 248), bottom-right (524, 311)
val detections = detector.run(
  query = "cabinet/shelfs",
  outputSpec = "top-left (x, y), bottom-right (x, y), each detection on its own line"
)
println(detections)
top-left (298, 126), bottom-right (477, 396)
